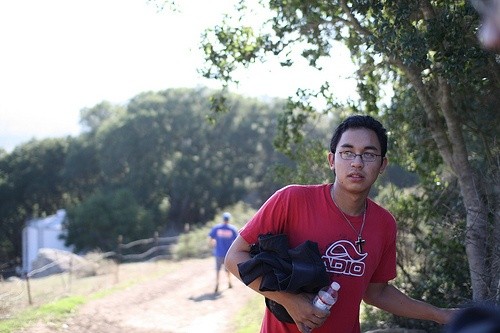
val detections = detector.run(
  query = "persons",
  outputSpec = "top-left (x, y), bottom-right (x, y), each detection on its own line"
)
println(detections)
top-left (207, 212), bottom-right (239, 293)
top-left (224, 116), bottom-right (467, 333)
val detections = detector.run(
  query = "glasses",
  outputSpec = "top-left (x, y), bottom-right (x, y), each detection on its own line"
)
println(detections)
top-left (335, 151), bottom-right (382, 161)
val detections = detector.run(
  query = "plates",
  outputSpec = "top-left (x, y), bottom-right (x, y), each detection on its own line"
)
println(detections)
top-left (304, 281), bottom-right (340, 331)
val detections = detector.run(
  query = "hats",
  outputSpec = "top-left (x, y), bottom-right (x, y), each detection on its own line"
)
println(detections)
top-left (223, 213), bottom-right (230, 219)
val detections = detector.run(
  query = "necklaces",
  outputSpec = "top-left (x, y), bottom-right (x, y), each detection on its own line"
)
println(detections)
top-left (331, 187), bottom-right (367, 254)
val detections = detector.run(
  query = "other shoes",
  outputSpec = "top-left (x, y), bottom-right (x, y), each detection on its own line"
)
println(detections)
top-left (228, 284), bottom-right (232, 288)
top-left (215, 289), bottom-right (218, 293)
